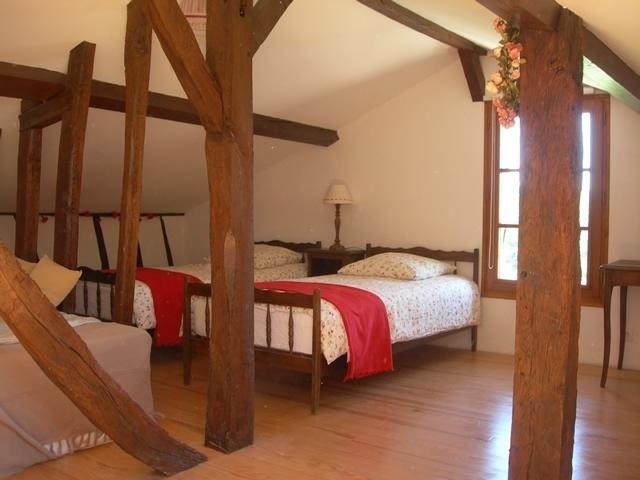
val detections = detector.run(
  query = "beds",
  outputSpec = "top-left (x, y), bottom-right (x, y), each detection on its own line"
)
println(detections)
top-left (1, 309), bottom-right (156, 480)
top-left (180, 242), bottom-right (483, 414)
top-left (60, 237), bottom-right (324, 354)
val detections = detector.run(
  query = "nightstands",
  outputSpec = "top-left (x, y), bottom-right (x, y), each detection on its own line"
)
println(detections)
top-left (306, 248), bottom-right (367, 278)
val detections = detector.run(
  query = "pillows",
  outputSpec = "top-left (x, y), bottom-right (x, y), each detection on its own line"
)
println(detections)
top-left (29, 253), bottom-right (83, 309)
top-left (334, 253), bottom-right (457, 283)
top-left (204, 244), bottom-right (304, 268)
top-left (14, 255), bottom-right (37, 277)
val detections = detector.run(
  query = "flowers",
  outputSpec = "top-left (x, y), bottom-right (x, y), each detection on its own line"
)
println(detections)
top-left (484, 9), bottom-right (527, 132)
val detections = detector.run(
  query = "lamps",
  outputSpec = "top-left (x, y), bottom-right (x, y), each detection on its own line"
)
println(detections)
top-left (323, 183), bottom-right (353, 255)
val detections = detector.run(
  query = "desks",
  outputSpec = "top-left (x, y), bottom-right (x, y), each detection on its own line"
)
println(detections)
top-left (598, 254), bottom-right (640, 393)
